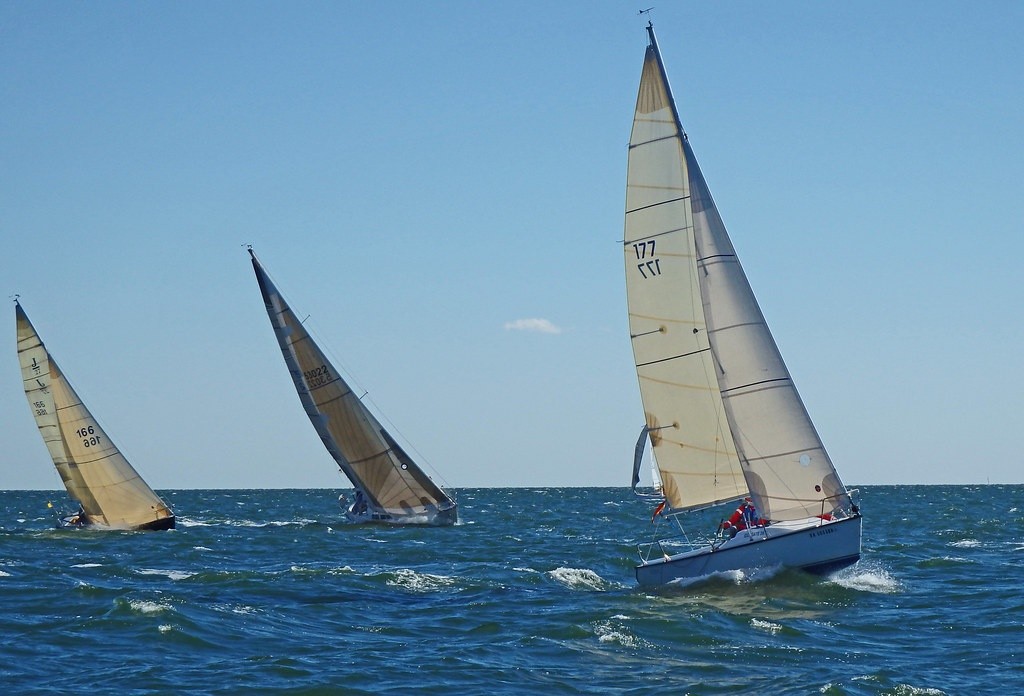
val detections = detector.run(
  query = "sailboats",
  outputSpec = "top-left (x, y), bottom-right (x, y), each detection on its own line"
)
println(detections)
top-left (9, 286), bottom-right (176, 533)
top-left (239, 238), bottom-right (462, 526)
top-left (616, 4), bottom-right (864, 590)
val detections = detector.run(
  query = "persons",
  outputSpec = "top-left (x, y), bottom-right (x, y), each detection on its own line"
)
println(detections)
top-left (722, 499), bottom-right (769, 537)
